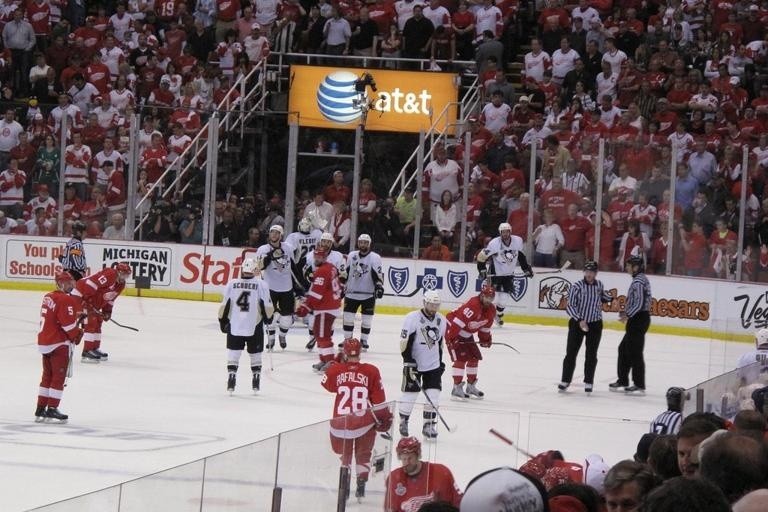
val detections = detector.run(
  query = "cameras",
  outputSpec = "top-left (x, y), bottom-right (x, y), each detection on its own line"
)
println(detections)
top-left (384, 202), bottom-right (393, 210)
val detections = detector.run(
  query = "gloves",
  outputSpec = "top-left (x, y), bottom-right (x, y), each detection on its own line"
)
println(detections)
top-left (478, 329), bottom-right (491, 347)
top-left (377, 413), bottom-right (392, 431)
top-left (296, 305), bottom-right (309, 317)
top-left (373, 287), bottom-right (384, 298)
top-left (403, 360), bottom-right (418, 383)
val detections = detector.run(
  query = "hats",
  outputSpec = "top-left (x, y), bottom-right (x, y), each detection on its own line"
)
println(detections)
top-left (251, 23), bottom-right (260, 30)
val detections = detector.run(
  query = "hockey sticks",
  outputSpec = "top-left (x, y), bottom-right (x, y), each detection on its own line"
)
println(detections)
top-left (487, 259), bottom-right (572, 277)
top-left (415, 378), bottom-right (458, 432)
top-left (367, 398), bottom-right (392, 440)
top-left (89, 303), bottom-right (139, 331)
top-left (63, 313), bottom-right (87, 387)
top-left (445, 341), bottom-right (518, 352)
top-left (345, 284), bottom-right (421, 297)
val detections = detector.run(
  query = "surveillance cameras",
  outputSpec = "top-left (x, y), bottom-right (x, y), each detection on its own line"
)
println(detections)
top-left (363, 72), bottom-right (377, 92)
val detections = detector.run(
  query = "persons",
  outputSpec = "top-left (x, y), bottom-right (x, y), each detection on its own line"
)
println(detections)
top-left (0, 0), bottom-right (768, 512)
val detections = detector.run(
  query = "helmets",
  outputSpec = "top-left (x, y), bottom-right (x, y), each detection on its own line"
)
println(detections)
top-left (298, 217), bottom-right (334, 257)
top-left (116, 263), bottom-right (131, 274)
top-left (241, 257), bottom-right (256, 274)
top-left (55, 270), bottom-right (71, 281)
top-left (357, 233), bottom-right (371, 247)
top-left (72, 220), bottom-right (87, 232)
top-left (481, 286), bottom-right (495, 297)
top-left (626, 255), bottom-right (643, 264)
top-left (396, 437), bottom-right (420, 453)
top-left (424, 291), bottom-right (440, 304)
top-left (666, 387), bottom-right (685, 405)
top-left (584, 262), bottom-right (598, 271)
top-left (270, 224), bottom-right (284, 235)
top-left (344, 338), bottom-right (360, 355)
top-left (498, 223), bottom-right (512, 234)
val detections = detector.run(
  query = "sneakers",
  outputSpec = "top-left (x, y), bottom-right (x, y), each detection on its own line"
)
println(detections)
top-left (360, 339), bottom-right (369, 348)
top-left (422, 426), bottom-right (437, 437)
top-left (399, 422), bottom-right (408, 436)
top-left (253, 377), bottom-right (259, 388)
top-left (452, 382), bottom-right (483, 397)
top-left (312, 362), bottom-right (327, 370)
top-left (228, 374), bottom-right (236, 387)
top-left (609, 382), bottom-right (645, 391)
top-left (559, 382), bottom-right (569, 389)
top-left (82, 349), bottom-right (107, 359)
top-left (36, 408), bottom-right (68, 419)
top-left (585, 383), bottom-right (592, 392)
top-left (266, 335), bottom-right (286, 347)
